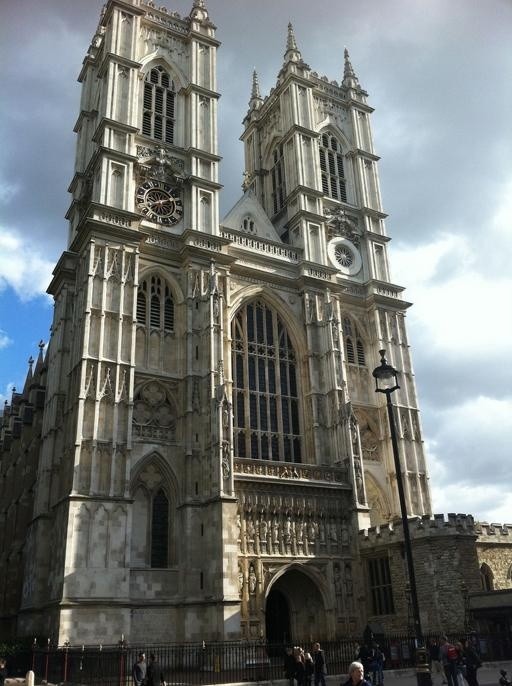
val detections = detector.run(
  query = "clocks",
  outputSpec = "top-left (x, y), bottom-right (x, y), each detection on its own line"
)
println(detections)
top-left (135, 181), bottom-right (184, 226)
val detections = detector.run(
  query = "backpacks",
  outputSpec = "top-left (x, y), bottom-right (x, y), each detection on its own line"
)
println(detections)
top-left (447, 644), bottom-right (459, 661)
top-left (416, 651), bottom-right (429, 664)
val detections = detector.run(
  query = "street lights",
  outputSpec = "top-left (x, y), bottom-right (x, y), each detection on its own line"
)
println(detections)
top-left (373, 349), bottom-right (434, 686)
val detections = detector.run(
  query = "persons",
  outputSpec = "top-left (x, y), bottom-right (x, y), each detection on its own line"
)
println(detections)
top-left (142, 653), bottom-right (167, 686)
top-left (133, 653), bottom-right (147, 686)
top-left (0, 658), bottom-right (10, 686)
top-left (282, 634), bottom-right (485, 686)
